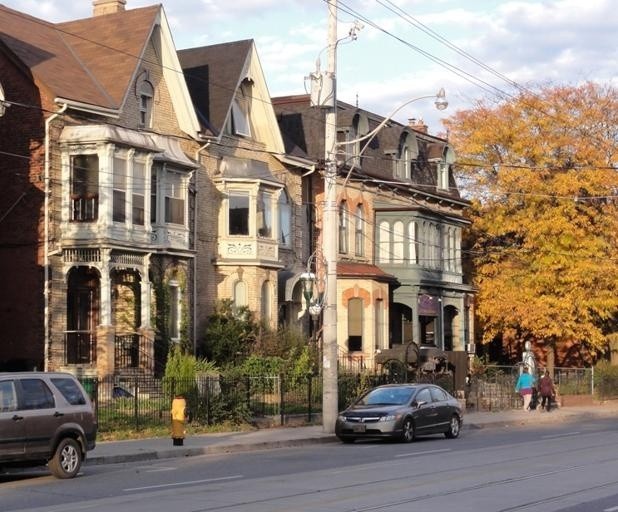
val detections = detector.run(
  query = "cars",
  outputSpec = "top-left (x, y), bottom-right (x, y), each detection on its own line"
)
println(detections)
top-left (330, 381), bottom-right (469, 442)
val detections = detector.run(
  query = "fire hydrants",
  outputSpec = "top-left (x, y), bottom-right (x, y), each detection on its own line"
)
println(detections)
top-left (168, 392), bottom-right (188, 450)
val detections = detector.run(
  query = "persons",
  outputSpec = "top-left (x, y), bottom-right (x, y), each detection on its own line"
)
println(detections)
top-left (515, 367), bottom-right (536, 411)
top-left (539, 370), bottom-right (556, 411)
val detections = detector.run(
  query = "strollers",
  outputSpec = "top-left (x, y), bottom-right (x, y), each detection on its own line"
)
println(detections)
top-left (530, 376), bottom-right (562, 413)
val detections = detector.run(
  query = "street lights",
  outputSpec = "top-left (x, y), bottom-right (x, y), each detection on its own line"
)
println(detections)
top-left (311, 84), bottom-right (451, 431)
top-left (295, 262), bottom-right (324, 427)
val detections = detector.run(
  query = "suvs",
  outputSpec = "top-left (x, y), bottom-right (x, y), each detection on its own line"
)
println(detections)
top-left (0, 372), bottom-right (100, 479)
